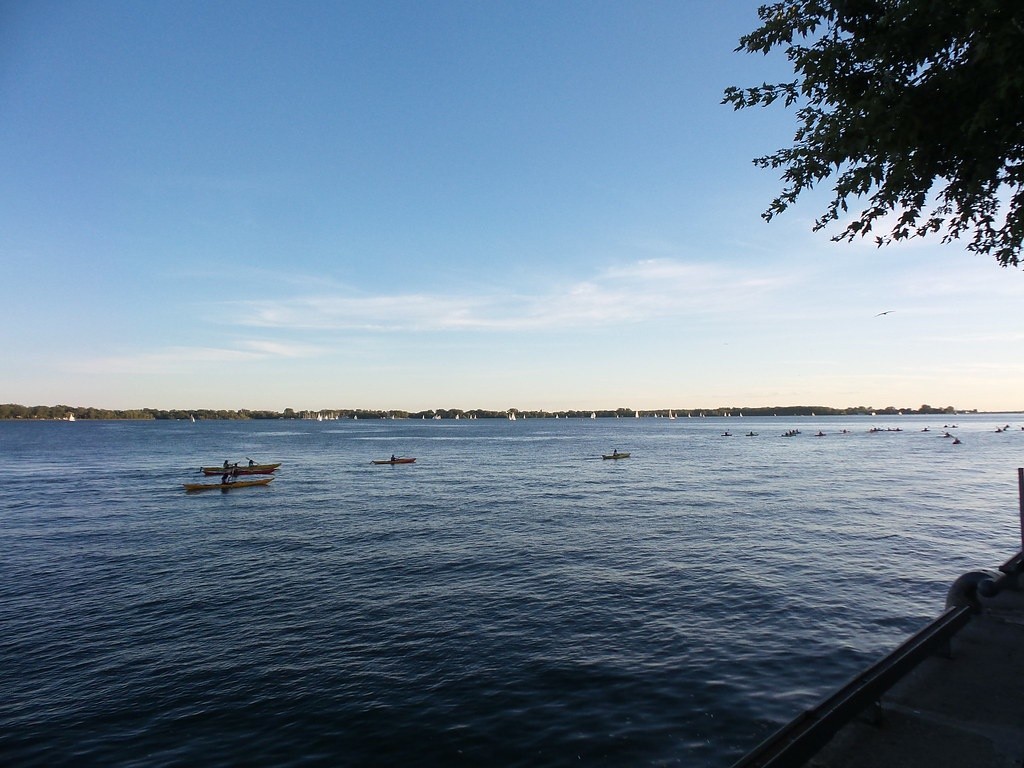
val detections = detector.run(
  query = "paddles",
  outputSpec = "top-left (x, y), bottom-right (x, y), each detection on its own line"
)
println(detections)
top-left (245, 456), bottom-right (260, 465)
top-left (229, 460), bottom-right (241, 466)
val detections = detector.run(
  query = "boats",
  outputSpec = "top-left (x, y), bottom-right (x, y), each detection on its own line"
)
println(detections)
top-left (602, 453), bottom-right (631, 460)
top-left (182, 477), bottom-right (275, 490)
top-left (746, 434), bottom-right (759, 436)
top-left (781, 432), bottom-right (801, 437)
top-left (200, 463), bottom-right (281, 476)
top-left (372, 457), bottom-right (417, 464)
top-left (721, 434), bottom-right (732, 436)
top-left (815, 434), bottom-right (826, 436)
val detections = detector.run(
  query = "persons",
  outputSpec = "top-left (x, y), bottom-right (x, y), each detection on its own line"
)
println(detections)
top-left (223, 460), bottom-right (238, 469)
top-left (222, 472), bottom-right (229, 485)
top-left (249, 459), bottom-right (254, 466)
top-left (614, 449), bottom-right (619, 456)
top-left (391, 454), bottom-right (394, 461)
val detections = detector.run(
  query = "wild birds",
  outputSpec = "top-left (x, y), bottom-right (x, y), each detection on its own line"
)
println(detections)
top-left (875, 311), bottom-right (894, 317)
